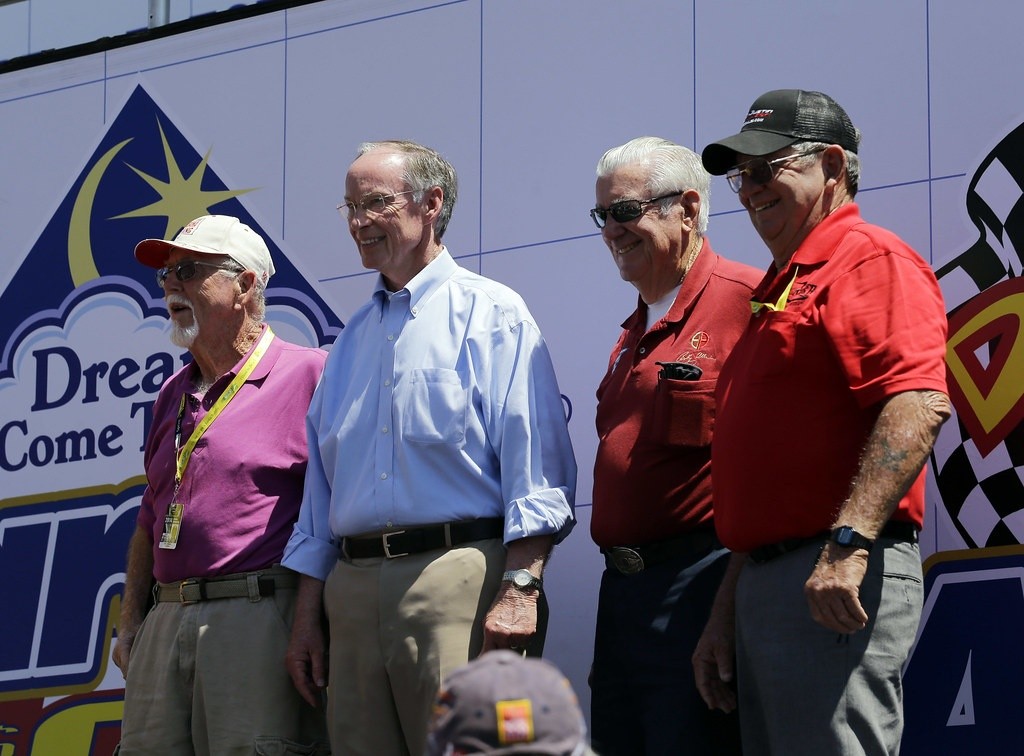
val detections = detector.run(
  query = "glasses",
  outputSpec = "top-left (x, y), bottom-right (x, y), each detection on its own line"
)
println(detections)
top-left (337, 187), bottom-right (424, 220)
top-left (590, 191), bottom-right (683, 229)
top-left (156, 262), bottom-right (243, 288)
top-left (726, 147), bottom-right (826, 193)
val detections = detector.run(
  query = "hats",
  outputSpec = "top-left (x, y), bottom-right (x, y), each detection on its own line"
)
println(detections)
top-left (701, 89), bottom-right (860, 178)
top-left (429, 649), bottom-right (587, 756)
top-left (133, 214), bottom-right (276, 289)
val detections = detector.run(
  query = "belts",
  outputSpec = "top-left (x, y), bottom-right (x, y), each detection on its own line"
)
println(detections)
top-left (155, 577), bottom-right (266, 604)
top-left (601, 537), bottom-right (696, 573)
top-left (748, 521), bottom-right (920, 563)
top-left (337, 526), bottom-right (494, 563)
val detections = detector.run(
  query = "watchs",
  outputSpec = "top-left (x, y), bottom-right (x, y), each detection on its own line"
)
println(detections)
top-left (826, 525), bottom-right (874, 552)
top-left (502, 569), bottom-right (542, 590)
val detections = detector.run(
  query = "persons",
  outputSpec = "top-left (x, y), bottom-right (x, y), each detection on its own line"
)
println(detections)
top-left (591, 134), bottom-right (767, 756)
top-left (281, 140), bottom-right (578, 756)
top-left (111, 212), bottom-right (329, 755)
top-left (691, 88), bottom-right (952, 756)
